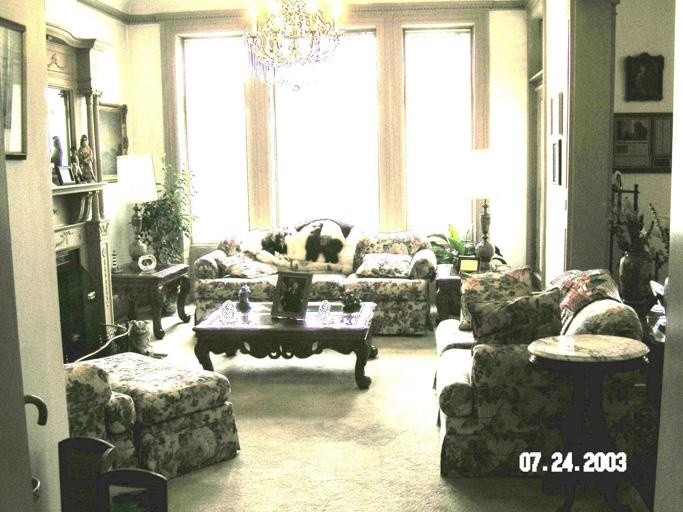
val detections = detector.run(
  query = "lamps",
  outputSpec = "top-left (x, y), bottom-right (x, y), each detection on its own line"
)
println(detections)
top-left (460, 148), bottom-right (504, 271)
top-left (239, 0), bottom-right (351, 93)
top-left (115, 152), bottom-right (158, 269)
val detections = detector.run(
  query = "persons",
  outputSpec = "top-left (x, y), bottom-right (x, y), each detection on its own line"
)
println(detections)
top-left (77, 135), bottom-right (94, 182)
top-left (50, 136), bottom-right (62, 185)
top-left (280, 280), bottom-right (301, 312)
top-left (71, 147), bottom-right (83, 185)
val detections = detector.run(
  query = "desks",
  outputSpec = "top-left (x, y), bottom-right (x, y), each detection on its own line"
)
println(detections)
top-left (522, 332), bottom-right (649, 509)
top-left (431, 262), bottom-right (462, 325)
top-left (111, 261), bottom-right (191, 338)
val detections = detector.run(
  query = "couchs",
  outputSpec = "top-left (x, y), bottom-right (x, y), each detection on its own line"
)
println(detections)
top-left (189, 219), bottom-right (437, 338)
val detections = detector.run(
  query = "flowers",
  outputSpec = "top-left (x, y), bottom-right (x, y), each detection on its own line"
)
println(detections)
top-left (604, 165), bottom-right (668, 305)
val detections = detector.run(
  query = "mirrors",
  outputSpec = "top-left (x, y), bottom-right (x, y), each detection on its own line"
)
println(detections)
top-left (45, 86), bottom-right (74, 178)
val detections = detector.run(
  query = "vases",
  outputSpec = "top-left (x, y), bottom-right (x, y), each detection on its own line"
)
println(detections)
top-left (616, 251), bottom-right (651, 308)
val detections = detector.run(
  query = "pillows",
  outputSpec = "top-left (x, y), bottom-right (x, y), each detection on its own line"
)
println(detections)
top-left (465, 287), bottom-right (562, 345)
top-left (218, 253), bottom-right (277, 279)
top-left (353, 252), bottom-right (412, 279)
top-left (457, 262), bottom-right (532, 331)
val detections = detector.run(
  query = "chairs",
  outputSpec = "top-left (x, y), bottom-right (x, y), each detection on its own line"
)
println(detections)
top-left (62, 351), bottom-right (240, 480)
top-left (432, 266), bottom-right (642, 480)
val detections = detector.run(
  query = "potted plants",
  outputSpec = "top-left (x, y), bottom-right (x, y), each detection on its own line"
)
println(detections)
top-left (132, 158), bottom-right (198, 315)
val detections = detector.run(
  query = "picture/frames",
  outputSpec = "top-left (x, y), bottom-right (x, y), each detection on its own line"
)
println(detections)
top-left (270, 270), bottom-right (314, 320)
top-left (98, 102), bottom-right (130, 184)
top-left (610, 112), bottom-right (671, 174)
top-left (137, 255), bottom-right (157, 271)
top-left (544, 92), bottom-right (562, 186)
top-left (0, 16), bottom-right (30, 161)
top-left (56, 164), bottom-right (76, 185)
top-left (623, 51), bottom-right (663, 101)
